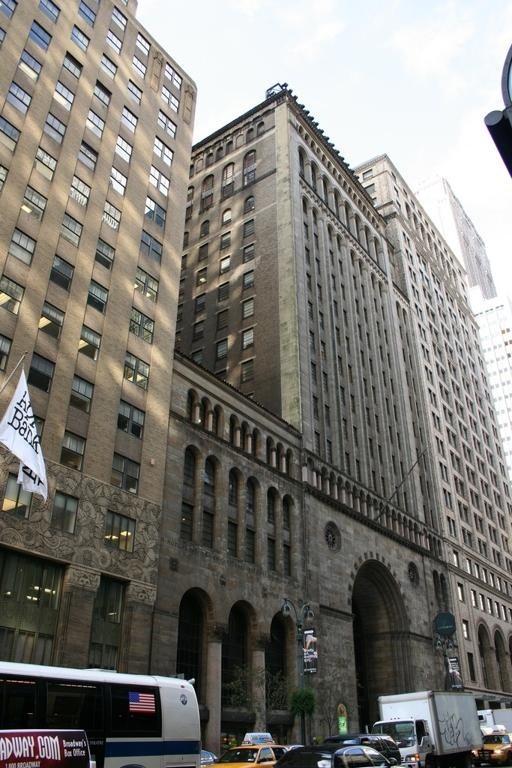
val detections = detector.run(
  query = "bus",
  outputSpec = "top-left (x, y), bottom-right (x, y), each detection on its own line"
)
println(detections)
top-left (0, 661), bottom-right (202, 767)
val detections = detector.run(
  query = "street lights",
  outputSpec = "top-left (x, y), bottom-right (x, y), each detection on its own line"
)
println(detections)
top-left (282, 598), bottom-right (315, 745)
top-left (436, 639), bottom-right (452, 690)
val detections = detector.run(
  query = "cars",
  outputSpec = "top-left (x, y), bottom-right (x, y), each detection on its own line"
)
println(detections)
top-left (201, 731), bottom-right (401, 768)
top-left (472, 729), bottom-right (511, 766)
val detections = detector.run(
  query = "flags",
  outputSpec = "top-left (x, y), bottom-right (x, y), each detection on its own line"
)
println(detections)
top-left (128, 690), bottom-right (157, 713)
top-left (0, 362), bottom-right (47, 507)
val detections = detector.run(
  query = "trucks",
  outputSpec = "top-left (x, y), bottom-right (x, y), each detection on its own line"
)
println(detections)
top-left (371, 690), bottom-right (484, 768)
top-left (477, 707), bottom-right (512, 733)
